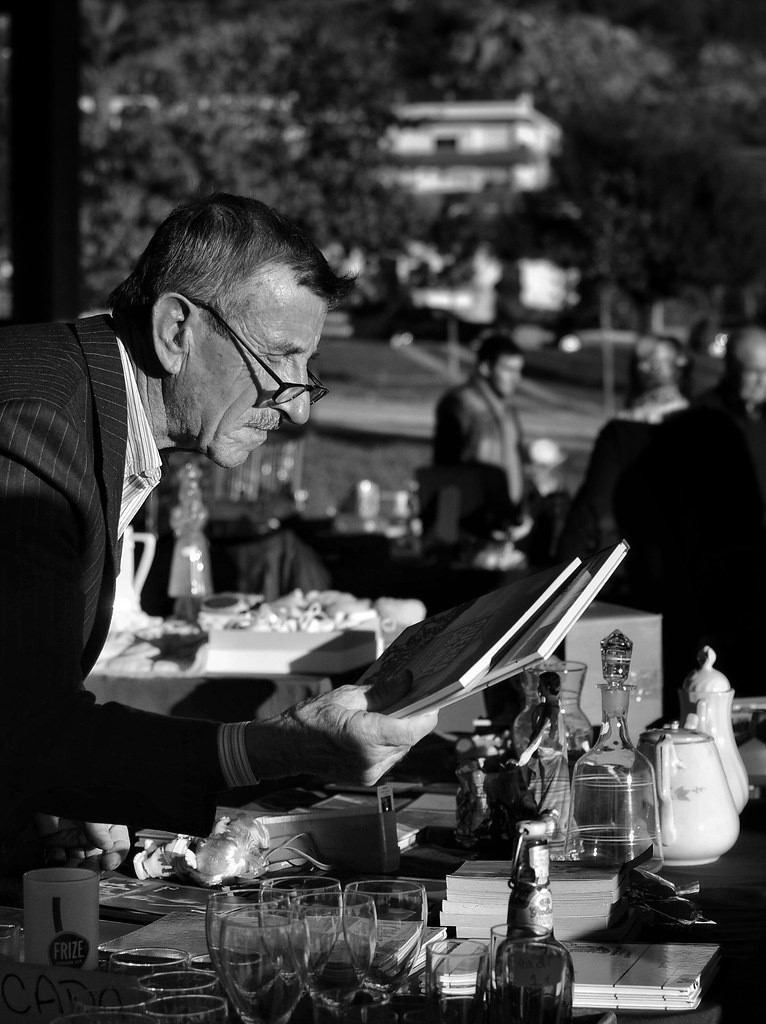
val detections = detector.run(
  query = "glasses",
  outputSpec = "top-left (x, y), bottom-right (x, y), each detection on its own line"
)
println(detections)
top-left (187, 297), bottom-right (330, 406)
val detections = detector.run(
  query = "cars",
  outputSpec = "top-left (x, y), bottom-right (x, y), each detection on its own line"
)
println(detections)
top-left (159, 327), bottom-right (672, 552)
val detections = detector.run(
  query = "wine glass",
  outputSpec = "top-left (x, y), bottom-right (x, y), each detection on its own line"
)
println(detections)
top-left (206, 876), bottom-right (428, 1024)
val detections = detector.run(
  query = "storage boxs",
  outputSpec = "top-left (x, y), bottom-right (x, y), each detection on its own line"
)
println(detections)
top-left (560, 601), bottom-right (663, 759)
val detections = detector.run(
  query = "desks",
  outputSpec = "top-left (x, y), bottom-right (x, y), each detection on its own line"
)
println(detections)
top-left (82, 612), bottom-right (385, 722)
top-left (0, 697), bottom-right (766, 1024)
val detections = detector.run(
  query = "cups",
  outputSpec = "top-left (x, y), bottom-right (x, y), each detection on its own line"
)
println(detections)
top-left (514, 662), bottom-right (595, 797)
top-left (52, 947), bottom-right (263, 1024)
top-left (431, 939), bottom-right (489, 1024)
top-left (23, 867), bottom-right (101, 969)
top-left (491, 922), bottom-right (551, 982)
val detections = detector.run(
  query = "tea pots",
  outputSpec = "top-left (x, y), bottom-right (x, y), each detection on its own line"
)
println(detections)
top-left (633, 720), bottom-right (740, 869)
top-left (674, 645), bottom-right (750, 823)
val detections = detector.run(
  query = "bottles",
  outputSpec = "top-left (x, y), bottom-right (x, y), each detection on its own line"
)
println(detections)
top-left (569, 631), bottom-right (656, 869)
top-left (492, 820), bottom-right (574, 1024)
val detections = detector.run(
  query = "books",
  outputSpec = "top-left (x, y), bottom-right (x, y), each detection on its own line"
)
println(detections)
top-left (357, 536), bottom-right (631, 713)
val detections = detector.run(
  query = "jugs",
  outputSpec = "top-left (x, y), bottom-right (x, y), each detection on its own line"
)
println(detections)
top-left (109, 526), bottom-right (157, 634)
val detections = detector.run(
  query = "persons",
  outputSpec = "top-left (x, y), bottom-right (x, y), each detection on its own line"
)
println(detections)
top-left (514, 671), bottom-right (584, 861)
top-left (431, 325), bottom-right (766, 699)
top-left (1, 190), bottom-right (439, 875)
